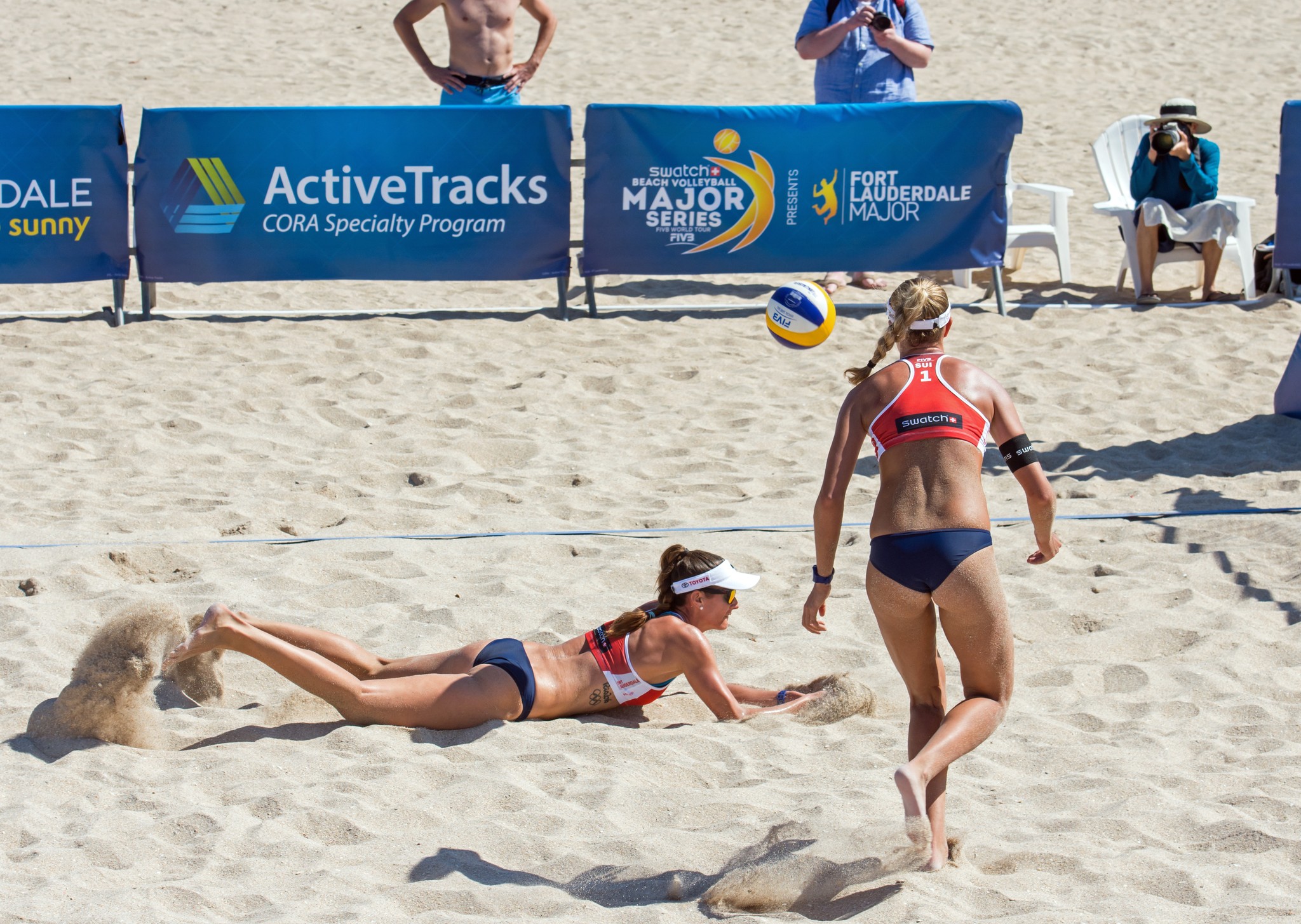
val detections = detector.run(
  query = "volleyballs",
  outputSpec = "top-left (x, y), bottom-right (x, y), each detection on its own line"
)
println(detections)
top-left (766, 280), bottom-right (836, 350)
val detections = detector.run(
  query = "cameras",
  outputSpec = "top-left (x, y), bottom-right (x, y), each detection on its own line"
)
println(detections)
top-left (868, 11), bottom-right (890, 31)
top-left (1150, 122), bottom-right (1182, 153)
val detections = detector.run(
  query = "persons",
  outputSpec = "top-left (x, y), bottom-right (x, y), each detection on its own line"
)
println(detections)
top-left (793, 0), bottom-right (935, 294)
top-left (800, 275), bottom-right (1061, 875)
top-left (393, 0), bottom-right (558, 106)
top-left (175, 546), bottom-right (811, 732)
top-left (1129, 97), bottom-right (1240, 306)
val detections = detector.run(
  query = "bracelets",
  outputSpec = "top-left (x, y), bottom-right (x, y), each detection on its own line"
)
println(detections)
top-left (776, 691), bottom-right (786, 705)
top-left (812, 565), bottom-right (835, 584)
top-left (999, 432), bottom-right (1040, 475)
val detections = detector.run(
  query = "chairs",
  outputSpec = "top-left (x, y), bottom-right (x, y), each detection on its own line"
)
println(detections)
top-left (1090, 115), bottom-right (1256, 299)
top-left (952, 155), bottom-right (1074, 288)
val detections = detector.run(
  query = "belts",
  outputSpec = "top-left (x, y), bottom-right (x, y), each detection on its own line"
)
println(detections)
top-left (451, 74), bottom-right (516, 94)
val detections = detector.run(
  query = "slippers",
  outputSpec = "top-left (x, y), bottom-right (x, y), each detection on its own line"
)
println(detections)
top-left (1205, 291), bottom-right (1240, 302)
top-left (851, 274), bottom-right (890, 290)
top-left (1136, 293), bottom-right (1161, 304)
top-left (818, 279), bottom-right (847, 296)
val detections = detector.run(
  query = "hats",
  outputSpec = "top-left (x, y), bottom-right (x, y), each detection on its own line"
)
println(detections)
top-left (886, 297), bottom-right (951, 330)
top-left (671, 560), bottom-right (760, 595)
top-left (1144, 97), bottom-right (1212, 134)
